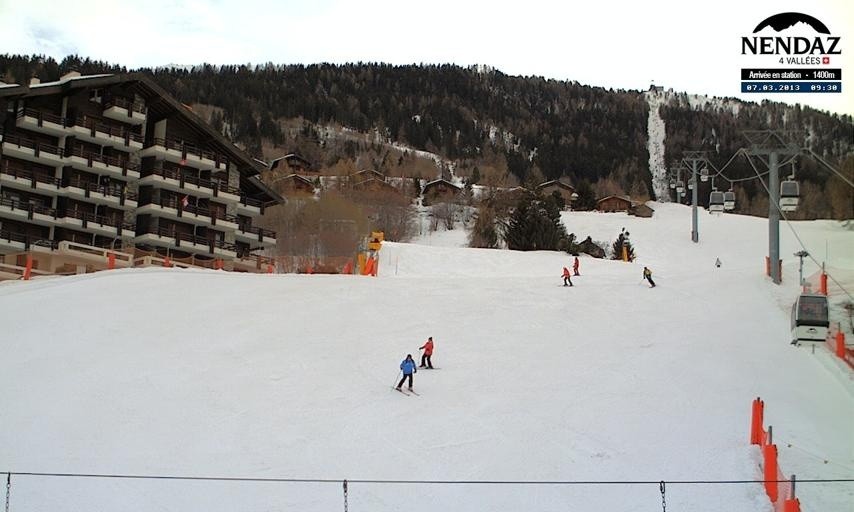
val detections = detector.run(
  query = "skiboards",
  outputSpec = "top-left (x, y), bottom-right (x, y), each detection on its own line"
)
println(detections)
top-left (392, 386), bottom-right (421, 396)
top-left (416, 367), bottom-right (442, 369)
top-left (558, 285), bottom-right (575, 287)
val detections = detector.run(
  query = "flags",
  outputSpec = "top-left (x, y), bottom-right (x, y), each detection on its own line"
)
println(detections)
top-left (182, 196), bottom-right (189, 207)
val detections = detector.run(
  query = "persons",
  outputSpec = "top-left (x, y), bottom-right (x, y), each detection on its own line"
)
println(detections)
top-left (714, 258), bottom-right (722, 268)
top-left (395, 354), bottom-right (417, 392)
top-left (572, 256), bottom-right (579, 275)
top-left (560, 266), bottom-right (573, 286)
top-left (418, 336), bottom-right (434, 370)
top-left (643, 266), bottom-right (656, 287)
